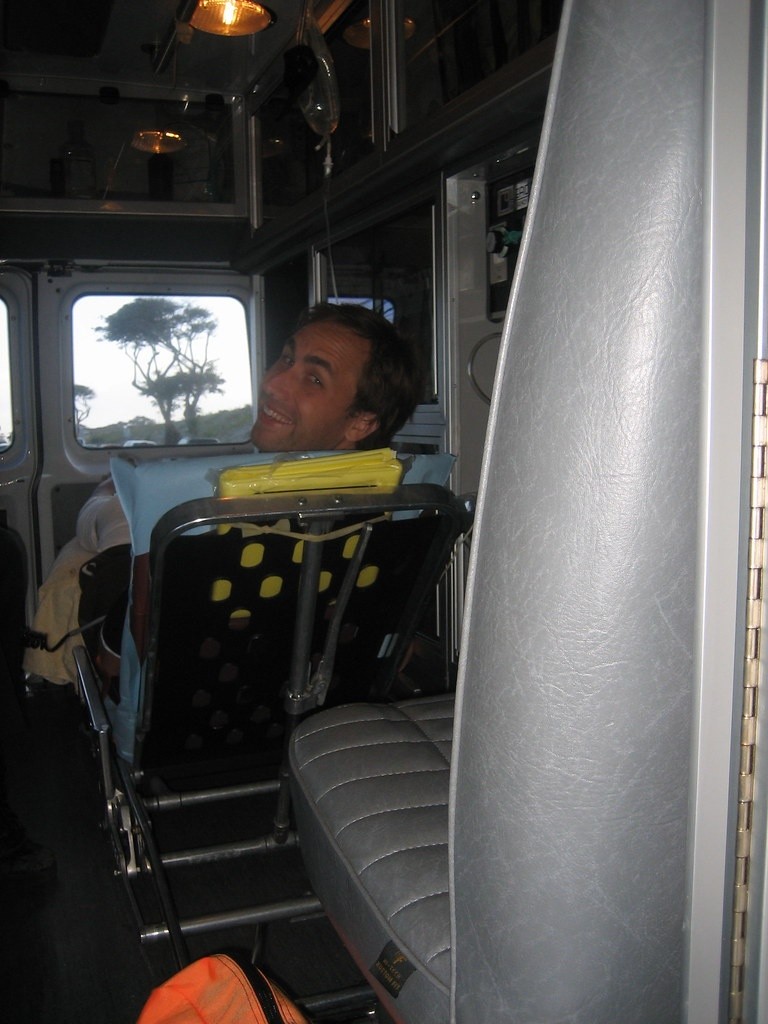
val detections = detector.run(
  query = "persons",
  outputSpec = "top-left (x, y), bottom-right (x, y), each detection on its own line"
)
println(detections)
top-left (76, 304), bottom-right (423, 726)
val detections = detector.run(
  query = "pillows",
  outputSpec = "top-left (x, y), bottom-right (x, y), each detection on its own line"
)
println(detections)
top-left (109, 450), bottom-right (460, 712)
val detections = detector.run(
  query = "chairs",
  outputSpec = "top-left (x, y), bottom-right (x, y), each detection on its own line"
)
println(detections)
top-left (72, 484), bottom-right (468, 1017)
top-left (285, 0), bottom-right (768, 1024)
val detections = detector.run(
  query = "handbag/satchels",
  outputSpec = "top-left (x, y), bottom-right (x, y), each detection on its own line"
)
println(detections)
top-left (135, 953), bottom-right (313, 1024)
